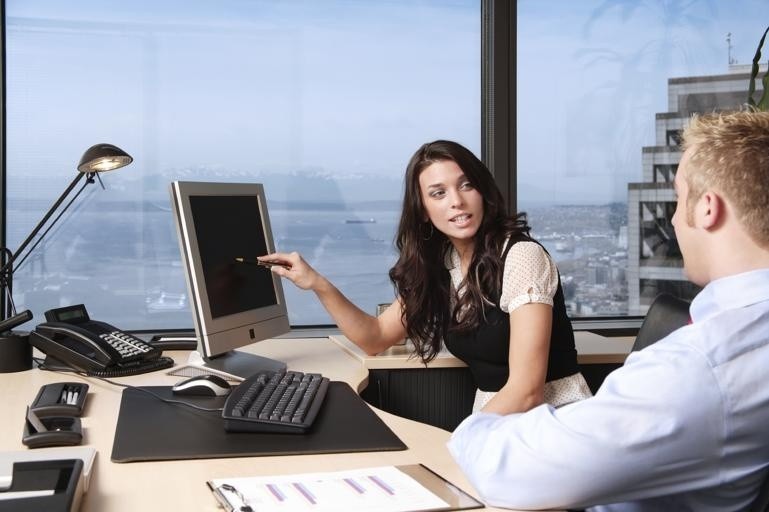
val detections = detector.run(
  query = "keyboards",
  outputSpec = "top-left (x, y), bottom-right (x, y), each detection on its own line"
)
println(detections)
top-left (222, 370), bottom-right (330, 434)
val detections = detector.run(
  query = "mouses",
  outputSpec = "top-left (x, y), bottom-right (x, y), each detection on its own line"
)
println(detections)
top-left (171, 375), bottom-right (232, 396)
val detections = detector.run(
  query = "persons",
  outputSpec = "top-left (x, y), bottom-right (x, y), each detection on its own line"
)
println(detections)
top-left (446, 106), bottom-right (769, 511)
top-left (256, 138), bottom-right (594, 415)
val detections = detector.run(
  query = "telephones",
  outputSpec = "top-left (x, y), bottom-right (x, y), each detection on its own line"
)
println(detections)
top-left (28, 304), bottom-right (162, 376)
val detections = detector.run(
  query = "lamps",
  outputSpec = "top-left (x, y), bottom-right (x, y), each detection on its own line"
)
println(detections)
top-left (2, 143), bottom-right (134, 373)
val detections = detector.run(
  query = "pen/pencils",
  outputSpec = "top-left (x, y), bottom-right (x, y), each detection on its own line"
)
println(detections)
top-left (235, 257), bottom-right (292, 271)
top-left (61, 386), bottom-right (79, 405)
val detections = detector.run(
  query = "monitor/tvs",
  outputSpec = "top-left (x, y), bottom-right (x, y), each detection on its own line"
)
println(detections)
top-left (169, 181), bottom-right (290, 382)
